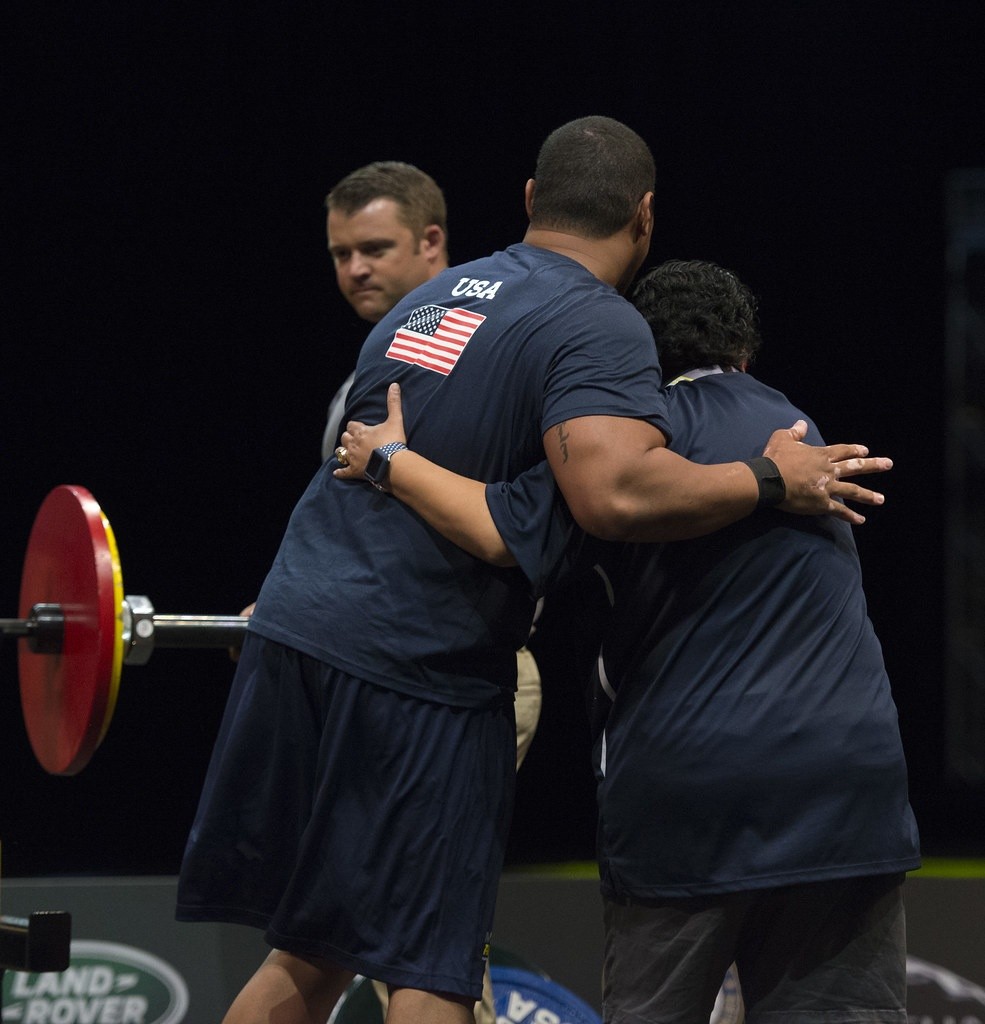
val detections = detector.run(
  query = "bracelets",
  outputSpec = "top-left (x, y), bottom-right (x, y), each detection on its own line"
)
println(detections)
top-left (742, 457), bottom-right (787, 510)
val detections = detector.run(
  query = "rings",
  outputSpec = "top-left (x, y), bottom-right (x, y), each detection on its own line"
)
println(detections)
top-left (337, 449), bottom-right (349, 464)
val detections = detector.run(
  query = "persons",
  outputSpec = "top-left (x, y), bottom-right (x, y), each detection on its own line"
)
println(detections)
top-left (332, 262), bottom-right (922, 1024)
top-left (176, 118), bottom-right (893, 1024)
top-left (229, 162), bottom-right (545, 1024)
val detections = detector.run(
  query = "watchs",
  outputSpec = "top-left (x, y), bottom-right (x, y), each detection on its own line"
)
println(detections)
top-left (363, 442), bottom-right (409, 492)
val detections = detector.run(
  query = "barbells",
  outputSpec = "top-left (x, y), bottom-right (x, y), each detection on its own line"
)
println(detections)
top-left (0, 484), bottom-right (250, 781)
